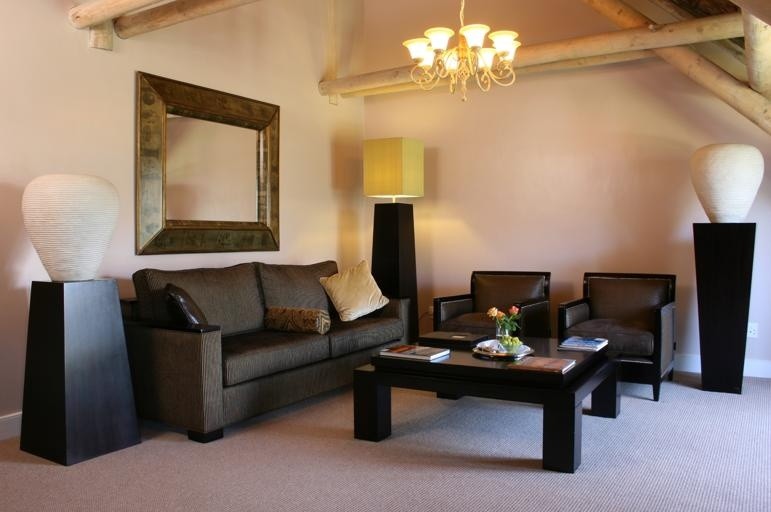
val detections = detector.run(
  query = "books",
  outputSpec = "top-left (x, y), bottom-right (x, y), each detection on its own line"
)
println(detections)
top-left (380, 343), bottom-right (451, 361)
top-left (556, 336), bottom-right (609, 352)
top-left (508, 356), bottom-right (576, 373)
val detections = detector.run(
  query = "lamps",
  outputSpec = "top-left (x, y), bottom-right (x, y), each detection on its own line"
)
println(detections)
top-left (362, 137), bottom-right (425, 204)
top-left (689, 143), bottom-right (764, 223)
top-left (20, 173), bottom-right (142, 282)
top-left (402, 0), bottom-right (522, 103)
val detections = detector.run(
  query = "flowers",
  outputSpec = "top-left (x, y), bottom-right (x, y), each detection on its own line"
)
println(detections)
top-left (489, 306), bottom-right (520, 331)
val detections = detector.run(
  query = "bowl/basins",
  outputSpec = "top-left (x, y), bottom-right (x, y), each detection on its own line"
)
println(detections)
top-left (497, 341), bottom-right (524, 354)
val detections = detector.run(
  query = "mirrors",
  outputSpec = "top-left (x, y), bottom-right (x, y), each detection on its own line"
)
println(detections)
top-left (135, 71), bottom-right (279, 255)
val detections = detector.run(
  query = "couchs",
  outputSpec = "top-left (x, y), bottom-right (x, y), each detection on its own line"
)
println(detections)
top-left (119, 294), bottom-right (410, 443)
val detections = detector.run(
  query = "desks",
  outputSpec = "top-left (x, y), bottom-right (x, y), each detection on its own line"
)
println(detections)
top-left (19, 281), bottom-right (142, 467)
top-left (355, 339), bottom-right (621, 473)
top-left (371, 203), bottom-right (419, 344)
top-left (693, 223), bottom-right (756, 395)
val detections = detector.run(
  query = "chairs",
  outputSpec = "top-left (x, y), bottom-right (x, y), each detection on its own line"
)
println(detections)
top-left (558, 273), bottom-right (676, 401)
top-left (434, 271), bottom-right (551, 338)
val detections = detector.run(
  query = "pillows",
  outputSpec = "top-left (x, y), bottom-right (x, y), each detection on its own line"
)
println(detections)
top-left (132, 263), bottom-right (266, 335)
top-left (264, 306), bottom-right (331, 335)
top-left (255, 261), bottom-right (338, 310)
top-left (162, 284), bottom-right (207, 327)
top-left (319, 258), bottom-right (389, 322)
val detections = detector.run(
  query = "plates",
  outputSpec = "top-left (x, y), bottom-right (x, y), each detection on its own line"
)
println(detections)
top-left (472, 344), bottom-right (535, 357)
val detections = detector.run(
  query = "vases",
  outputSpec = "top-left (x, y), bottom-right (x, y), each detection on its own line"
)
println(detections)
top-left (497, 327), bottom-right (510, 337)
